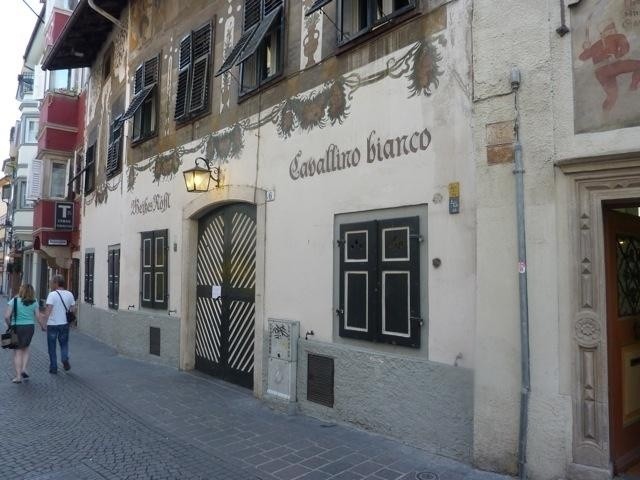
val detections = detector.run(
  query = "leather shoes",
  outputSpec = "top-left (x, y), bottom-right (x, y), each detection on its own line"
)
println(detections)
top-left (63, 361), bottom-right (71, 371)
top-left (49, 369), bottom-right (57, 374)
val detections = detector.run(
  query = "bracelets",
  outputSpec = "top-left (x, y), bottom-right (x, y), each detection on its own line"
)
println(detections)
top-left (38, 319), bottom-right (41, 323)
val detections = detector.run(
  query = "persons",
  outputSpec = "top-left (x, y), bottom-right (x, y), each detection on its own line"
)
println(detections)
top-left (42, 274), bottom-right (76, 373)
top-left (5, 283), bottom-right (42, 383)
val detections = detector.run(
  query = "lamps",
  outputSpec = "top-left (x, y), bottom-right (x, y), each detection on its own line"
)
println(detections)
top-left (183, 157), bottom-right (220, 192)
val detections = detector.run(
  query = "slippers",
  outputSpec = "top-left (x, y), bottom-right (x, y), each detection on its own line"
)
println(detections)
top-left (12, 377), bottom-right (21, 384)
top-left (20, 371), bottom-right (30, 378)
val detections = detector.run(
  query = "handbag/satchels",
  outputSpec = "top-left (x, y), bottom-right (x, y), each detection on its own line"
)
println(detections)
top-left (1, 325), bottom-right (19, 349)
top-left (66, 312), bottom-right (76, 323)
